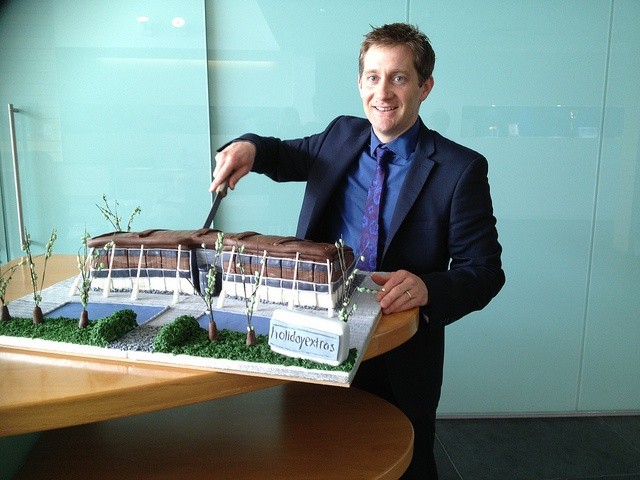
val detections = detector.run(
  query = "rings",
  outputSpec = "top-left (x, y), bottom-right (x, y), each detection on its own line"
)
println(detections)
top-left (406, 291), bottom-right (413, 299)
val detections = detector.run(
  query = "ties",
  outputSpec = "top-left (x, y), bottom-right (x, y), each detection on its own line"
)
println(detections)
top-left (357, 146), bottom-right (389, 272)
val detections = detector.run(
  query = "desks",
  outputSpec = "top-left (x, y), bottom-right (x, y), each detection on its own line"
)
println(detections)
top-left (1, 254), bottom-right (421, 437)
top-left (1, 381), bottom-right (416, 479)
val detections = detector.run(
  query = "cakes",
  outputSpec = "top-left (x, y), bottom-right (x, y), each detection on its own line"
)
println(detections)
top-left (0, 198), bottom-right (393, 390)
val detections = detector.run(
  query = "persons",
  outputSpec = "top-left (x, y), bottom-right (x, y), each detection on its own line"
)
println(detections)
top-left (207, 22), bottom-right (506, 480)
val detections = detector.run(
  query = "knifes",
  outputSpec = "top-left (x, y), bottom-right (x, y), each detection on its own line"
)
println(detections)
top-left (200, 173), bottom-right (231, 230)
